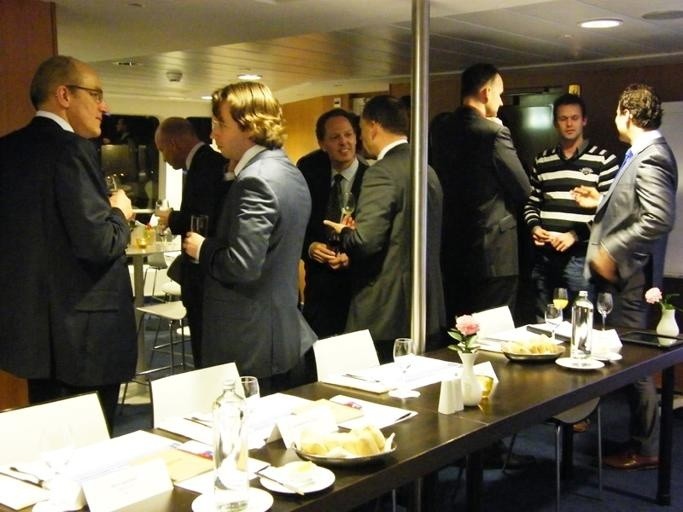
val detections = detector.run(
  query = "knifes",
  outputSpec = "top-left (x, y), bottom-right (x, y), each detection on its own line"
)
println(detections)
top-left (0, 471), bottom-right (50, 492)
top-left (254, 471), bottom-right (304, 497)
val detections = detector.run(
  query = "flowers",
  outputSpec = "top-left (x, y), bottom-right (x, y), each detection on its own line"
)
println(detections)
top-left (644, 286), bottom-right (681, 311)
top-left (449, 315), bottom-right (482, 352)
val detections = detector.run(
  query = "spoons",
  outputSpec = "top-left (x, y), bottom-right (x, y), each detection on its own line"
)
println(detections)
top-left (10, 467), bottom-right (46, 486)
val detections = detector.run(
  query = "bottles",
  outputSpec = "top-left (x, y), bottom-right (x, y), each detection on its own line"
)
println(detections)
top-left (570, 291), bottom-right (594, 361)
top-left (212, 378), bottom-right (249, 511)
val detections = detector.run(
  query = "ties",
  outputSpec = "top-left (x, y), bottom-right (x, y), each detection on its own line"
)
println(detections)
top-left (181, 170), bottom-right (189, 200)
top-left (325, 174), bottom-right (343, 224)
top-left (617, 148), bottom-right (633, 176)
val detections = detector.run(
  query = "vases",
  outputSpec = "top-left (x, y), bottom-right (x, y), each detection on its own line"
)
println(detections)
top-left (457, 351), bottom-right (483, 406)
top-left (656, 309), bottom-right (680, 348)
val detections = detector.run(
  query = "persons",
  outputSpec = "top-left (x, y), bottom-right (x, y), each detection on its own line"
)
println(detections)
top-left (428, 61), bottom-right (537, 470)
top-left (321, 94), bottom-right (447, 364)
top-left (153, 116), bottom-right (230, 368)
top-left (0, 54), bottom-right (139, 436)
top-left (569, 81), bottom-right (680, 471)
top-left (183, 82), bottom-right (320, 391)
top-left (522, 92), bottom-right (621, 432)
top-left (301, 108), bottom-right (371, 337)
top-left (102, 117), bottom-right (133, 144)
top-left (297, 111), bottom-right (361, 182)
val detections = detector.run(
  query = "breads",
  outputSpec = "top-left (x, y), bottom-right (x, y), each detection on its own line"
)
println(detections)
top-left (303, 424), bottom-right (387, 458)
top-left (505, 341), bottom-right (554, 355)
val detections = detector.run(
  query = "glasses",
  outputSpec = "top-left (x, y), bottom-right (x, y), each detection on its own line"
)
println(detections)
top-left (63, 84), bottom-right (104, 100)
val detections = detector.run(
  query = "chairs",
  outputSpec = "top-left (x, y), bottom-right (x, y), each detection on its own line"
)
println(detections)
top-left (117, 214), bottom-right (196, 429)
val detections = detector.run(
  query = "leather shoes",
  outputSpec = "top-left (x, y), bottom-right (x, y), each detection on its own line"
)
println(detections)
top-left (596, 450), bottom-right (659, 472)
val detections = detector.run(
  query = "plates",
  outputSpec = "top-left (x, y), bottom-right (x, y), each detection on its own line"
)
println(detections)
top-left (503, 348), bottom-right (564, 360)
top-left (291, 438), bottom-right (398, 465)
top-left (259, 463), bottom-right (337, 493)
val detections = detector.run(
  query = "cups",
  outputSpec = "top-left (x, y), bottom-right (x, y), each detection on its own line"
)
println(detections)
top-left (103, 174), bottom-right (121, 195)
top-left (328, 229), bottom-right (342, 250)
top-left (136, 238), bottom-right (149, 249)
top-left (475, 375), bottom-right (493, 398)
top-left (339, 192), bottom-right (356, 217)
top-left (237, 376), bottom-right (260, 408)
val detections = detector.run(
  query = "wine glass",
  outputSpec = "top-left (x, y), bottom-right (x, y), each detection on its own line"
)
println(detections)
top-left (597, 292), bottom-right (614, 330)
top-left (543, 287), bottom-right (569, 343)
top-left (190, 216), bottom-right (210, 264)
top-left (156, 200), bottom-right (170, 235)
top-left (388, 338), bottom-right (420, 398)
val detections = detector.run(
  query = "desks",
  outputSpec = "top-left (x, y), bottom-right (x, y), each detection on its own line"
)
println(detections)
top-left (1, 382), bottom-right (487, 512)
top-left (314, 317), bottom-right (683, 510)
